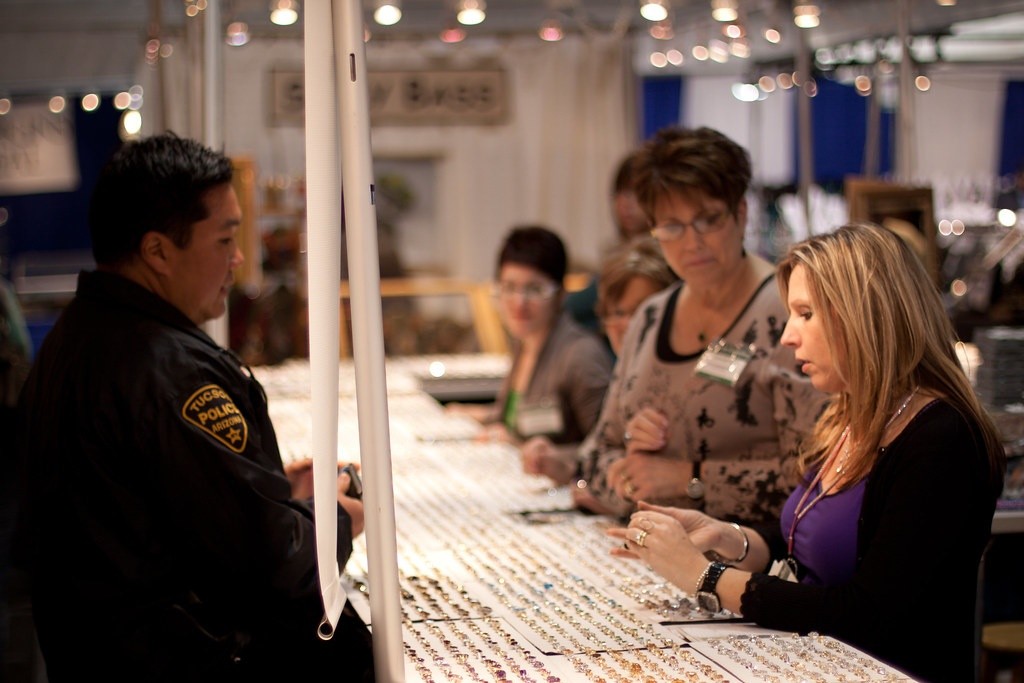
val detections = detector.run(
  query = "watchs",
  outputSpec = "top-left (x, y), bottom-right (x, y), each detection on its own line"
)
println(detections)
top-left (686, 461), bottom-right (704, 500)
top-left (696, 562), bottom-right (735, 616)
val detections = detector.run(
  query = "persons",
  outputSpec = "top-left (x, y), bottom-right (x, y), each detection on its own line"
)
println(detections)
top-left (606, 224), bottom-right (1005, 683)
top-left (690, 281), bottom-right (725, 342)
top-left (449, 223), bottom-right (612, 449)
top-left (0, 129), bottom-right (376, 683)
top-left (519, 126), bottom-right (835, 525)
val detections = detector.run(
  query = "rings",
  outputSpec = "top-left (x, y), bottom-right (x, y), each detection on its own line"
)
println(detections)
top-left (620, 472), bottom-right (629, 482)
top-left (637, 531), bottom-right (650, 547)
top-left (624, 432), bottom-right (631, 443)
top-left (638, 518), bottom-right (655, 531)
top-left (624, 480), bottom-right (635, 494)
top-left (250, 352), bottom-right (917, 683)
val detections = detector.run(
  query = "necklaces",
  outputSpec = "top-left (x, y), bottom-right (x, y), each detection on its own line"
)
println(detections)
top-left (835, 386), bottom-right (921, 474)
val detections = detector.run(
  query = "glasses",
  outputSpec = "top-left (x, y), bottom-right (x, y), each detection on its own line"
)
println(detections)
top-left (488, 281), bottom-right (552, 301)
top-left (643, 203), bottom-right (734, 241)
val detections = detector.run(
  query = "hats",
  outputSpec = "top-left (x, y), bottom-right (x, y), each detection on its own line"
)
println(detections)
top-left (495, 225), bottom-right (569, 288)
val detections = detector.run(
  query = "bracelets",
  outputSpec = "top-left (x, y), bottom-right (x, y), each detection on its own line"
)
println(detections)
top-left (692, 560), bottom-right (716, 598)
top-left (712, 523), bottom-right (749, 565)
